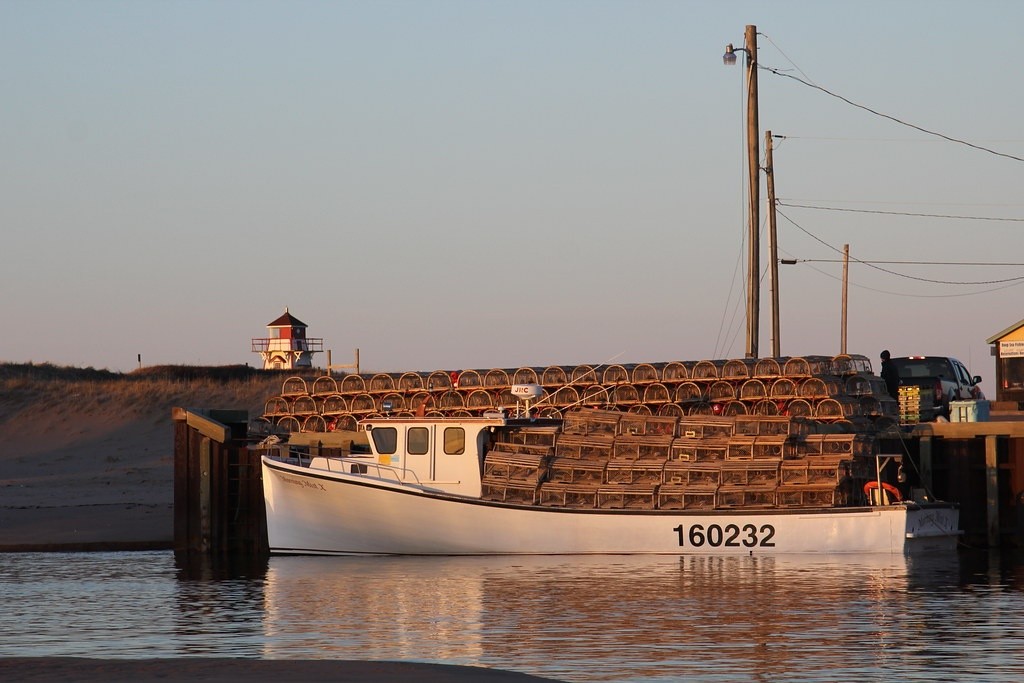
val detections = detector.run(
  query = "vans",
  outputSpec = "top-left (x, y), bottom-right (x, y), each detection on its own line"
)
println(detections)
top-left (878, 356), bottom-right (988, 416)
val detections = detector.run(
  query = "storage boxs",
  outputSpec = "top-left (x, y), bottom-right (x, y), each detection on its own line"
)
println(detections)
top-left (899, 385), bottom-right (933, 424)
top-left (949, 400), bottom-right (990, 422)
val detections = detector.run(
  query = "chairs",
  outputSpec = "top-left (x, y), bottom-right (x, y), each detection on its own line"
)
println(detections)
top-left (930, 364), bottom-right (943, 376)
top-left (905, 367), bottom-right (912, 377)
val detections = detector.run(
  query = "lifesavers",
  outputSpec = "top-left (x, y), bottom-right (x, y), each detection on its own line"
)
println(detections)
top-left (863, 481), bottom-right (904, 501)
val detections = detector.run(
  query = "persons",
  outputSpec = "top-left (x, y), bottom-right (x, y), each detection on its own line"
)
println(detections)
top-left (880, 350), bottom-right (898, 399)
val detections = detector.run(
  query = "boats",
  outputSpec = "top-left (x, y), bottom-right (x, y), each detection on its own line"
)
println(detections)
top-left (260, 416), bottom-right (967, 555)
